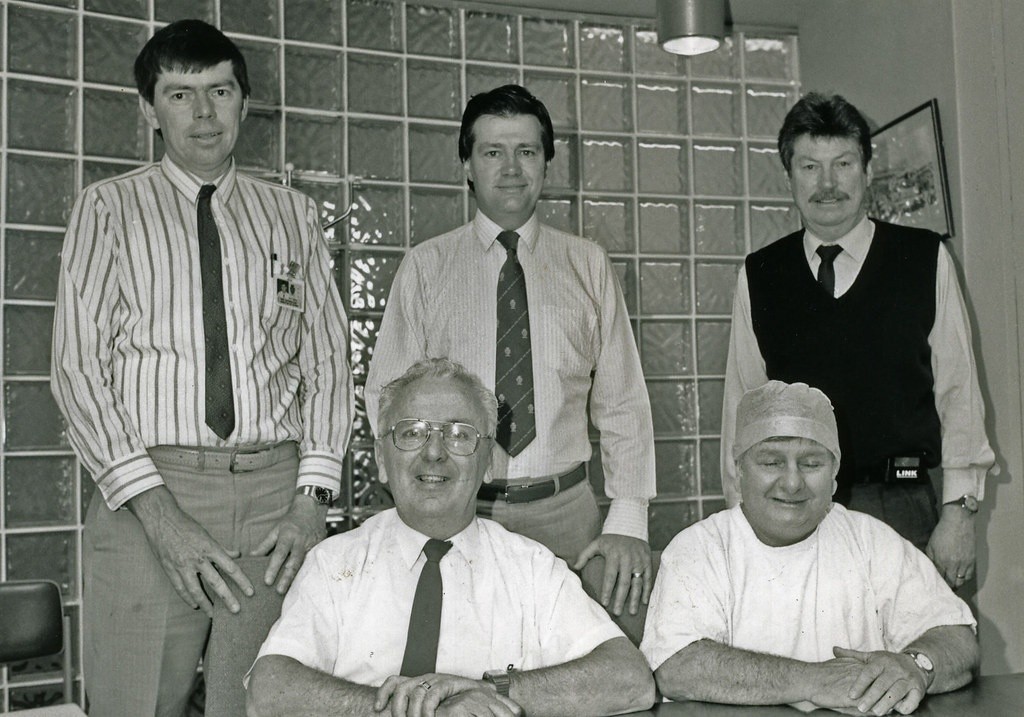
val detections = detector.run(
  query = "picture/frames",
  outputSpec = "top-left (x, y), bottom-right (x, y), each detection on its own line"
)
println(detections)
top-left (863, 98), bottom-right (955, 241)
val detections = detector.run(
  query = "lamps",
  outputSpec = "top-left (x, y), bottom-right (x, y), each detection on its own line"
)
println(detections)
top-left (655, 0), bottom-right (726, 57)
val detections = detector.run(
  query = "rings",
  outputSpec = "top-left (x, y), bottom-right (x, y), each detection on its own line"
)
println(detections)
top-left (419, 681), bottom-right (431, 691)
top-left (631, 572), bottom-right (644, 578)
top-left (956, 575), bottom-right (965, 578)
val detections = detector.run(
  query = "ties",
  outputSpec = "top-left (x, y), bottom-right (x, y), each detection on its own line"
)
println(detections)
top-left (495, 229), bottom-right (536, 459)
top-left (816, 244), bottom-right (844, 297)
top-left (196, 184), bottom-right (236, 441)
top-left (400, 539), bottom-right (454, 676)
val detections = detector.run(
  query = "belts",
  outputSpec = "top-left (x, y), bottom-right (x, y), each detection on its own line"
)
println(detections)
top-left (477, 461), bottom-right (587, 503)
top-left (147, 442), bottom-right (297, 474)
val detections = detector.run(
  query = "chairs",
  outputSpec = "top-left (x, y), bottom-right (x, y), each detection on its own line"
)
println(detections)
top-left (204, 555), bottom-right (286, 717)
top-left (561, 551), bottom-right (665, 652)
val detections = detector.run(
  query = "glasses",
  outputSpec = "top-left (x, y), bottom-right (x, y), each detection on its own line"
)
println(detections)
top-left (379, 418), bottom-right (492, 457)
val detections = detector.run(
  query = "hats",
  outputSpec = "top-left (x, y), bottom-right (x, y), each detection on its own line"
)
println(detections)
top-left (731, 379), bottom-right (841, 478)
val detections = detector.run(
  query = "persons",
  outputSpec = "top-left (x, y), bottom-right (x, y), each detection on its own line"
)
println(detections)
top-left (50, 21), bottom-right (356, 717)
top-left (243, 358), bottom-right (657, 717)
top-left (640, 380), bottom-right (982, 717)
top-left (720, 93), bottom-right (998, 592)
top-left (366, 85), bottom-right (655, 615)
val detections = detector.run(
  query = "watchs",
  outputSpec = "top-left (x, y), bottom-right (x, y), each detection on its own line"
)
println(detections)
top-left (903, 649), bottom-right (935, 688)
top-left (945, 495), bottom-right (980, 514)
top-left (297, 485), bottom-right (333, 508)
top-left (482, 670), bottom-right (510, 697)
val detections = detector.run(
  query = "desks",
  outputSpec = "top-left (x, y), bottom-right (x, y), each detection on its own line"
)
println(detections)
top-left (606, 672), bottom-right (1024, 717)
